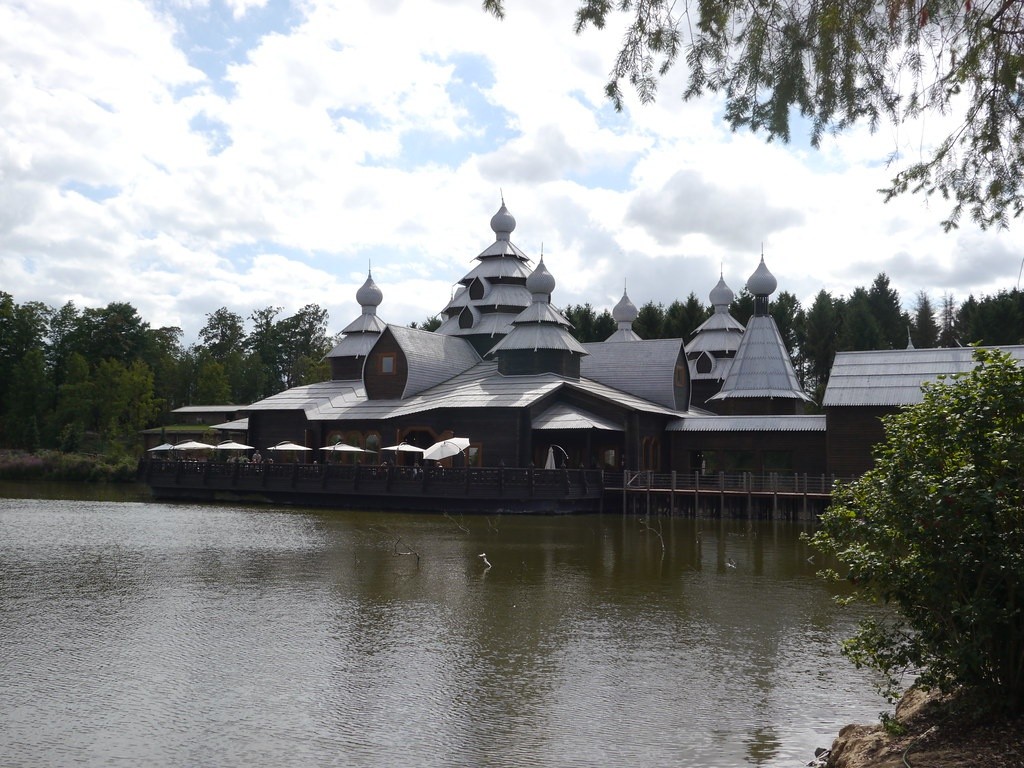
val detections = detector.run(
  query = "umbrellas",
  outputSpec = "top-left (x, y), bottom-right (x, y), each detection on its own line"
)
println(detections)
top-left (380, 442), bottom-right (425, 453)
top-left (544, 447), bottom-right (556, 469)
top-left (147, 440), bottom-right (254, 451)
top-left (319, 443), bottom-right (364, 452)
top-left (423, 437), bottom-right (470, 461)
top-left (267, 443), bottom-right (313, 451)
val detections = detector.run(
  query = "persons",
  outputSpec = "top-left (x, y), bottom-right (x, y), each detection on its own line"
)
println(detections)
top-left (252, 449), bottom-right (261, 463)
